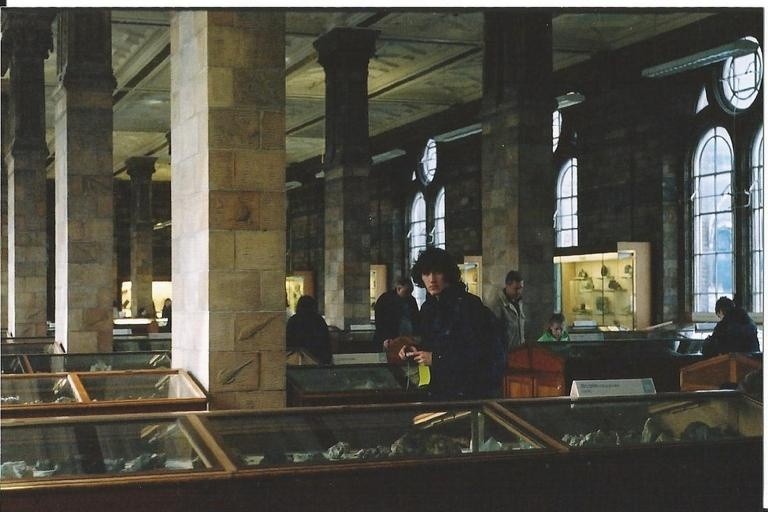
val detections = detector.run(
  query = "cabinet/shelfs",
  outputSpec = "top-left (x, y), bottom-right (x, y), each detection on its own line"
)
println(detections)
top-left (370, 265), bottom-right (387, 317)
top-left (284, 270), bottom-right (315, 314)
top-left (553, 250), bottom-right (651, 331)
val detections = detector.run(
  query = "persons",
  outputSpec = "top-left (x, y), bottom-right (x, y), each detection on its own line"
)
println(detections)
top-left (160, 297), bottom-right (171, 319)
top-left (701, 295), bottom-right (760, 358)
top-left (286, 294), bottom-right (332, 364)
top-left (536, 312), bottom-right (570, 342)
top-left (397, 246), bottom-right (496, 400)
top-left (135, 307), bottom-right (149, 319)
top-left (112, 299), bottom-right (122, 319)
top-left (487, 268), bottom-right (527, 346)
top-left (372, 276), bottom-right (418, 353)
